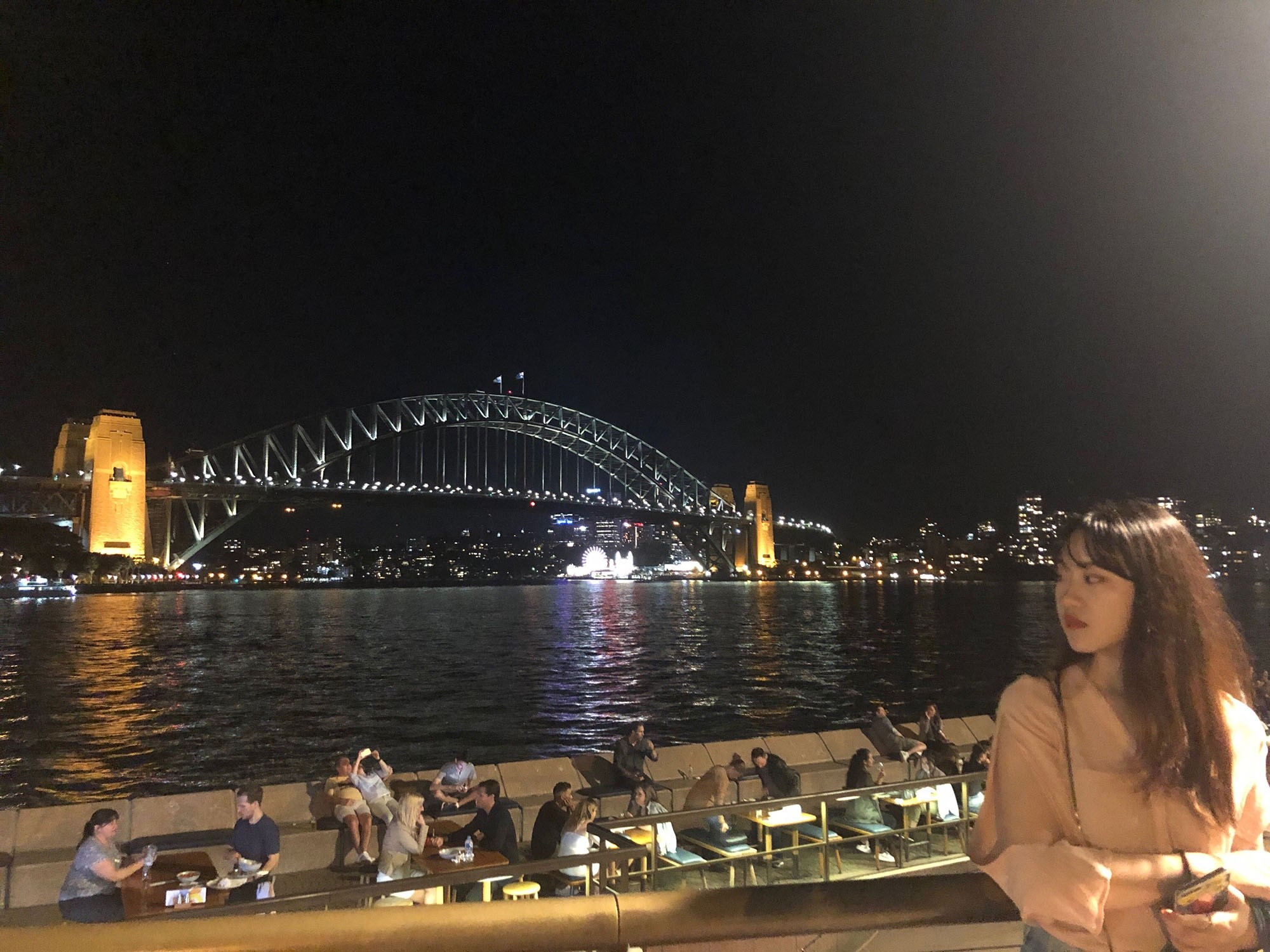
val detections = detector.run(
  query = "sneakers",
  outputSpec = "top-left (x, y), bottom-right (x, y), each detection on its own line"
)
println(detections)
top-left (856, 843), bottom-right (872, 854)
top-left (554, 885), bottom-right (579, 896)
top-left (355, 852), bottom-right (376, 867)
top-left (874, 852), bottom-right (895, 863)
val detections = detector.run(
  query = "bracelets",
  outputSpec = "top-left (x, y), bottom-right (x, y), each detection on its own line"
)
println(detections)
top-left (1170, 848), bottom-right (1193, 882)
top-left (650, 748), bottom-right (655, 751)
top-left (377, 758), bottom-right (382, 761)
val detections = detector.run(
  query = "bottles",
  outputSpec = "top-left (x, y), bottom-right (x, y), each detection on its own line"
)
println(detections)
top-left (185, 893), bottom-right (189, 903)
top-left (464, 836), bottom-right (474, 862)
top-left (688, 765), bottom-right (693, 779)
top-left (177, 894), bottom-right (182, 904)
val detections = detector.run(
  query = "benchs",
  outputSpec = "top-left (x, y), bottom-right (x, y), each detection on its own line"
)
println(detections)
top-left (1, 716), bottom-right (995, 911)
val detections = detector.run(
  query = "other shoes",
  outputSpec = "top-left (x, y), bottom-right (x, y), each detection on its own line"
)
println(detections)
top-left (441, 801), bottom-right (457, 812)
top-left (900, 750), bottom-right (910, 762)
top-left (913, 751), bottom-right (922, 759)
top-left (709, 865), bottom-right (729, 873)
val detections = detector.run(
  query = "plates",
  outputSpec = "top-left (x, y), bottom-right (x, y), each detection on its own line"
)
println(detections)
top-left (207, 876), bottom-right (247, 889)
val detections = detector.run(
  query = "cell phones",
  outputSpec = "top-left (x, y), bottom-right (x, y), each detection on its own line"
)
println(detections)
top-left (360, 748), bottom-right (372, 757)
top-left (1174, 867), bottom-right (1231, 916)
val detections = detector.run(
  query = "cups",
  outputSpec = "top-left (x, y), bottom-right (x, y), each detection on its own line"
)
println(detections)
top-left (144, 845), bottom-right (157, 866)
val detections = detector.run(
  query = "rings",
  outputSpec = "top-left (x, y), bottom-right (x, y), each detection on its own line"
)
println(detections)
top-left (1196, 913), bottom-right (1212, 930)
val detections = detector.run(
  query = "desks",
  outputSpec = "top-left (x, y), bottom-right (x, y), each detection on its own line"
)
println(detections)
top-left (732, 807), bottom-right (817, 884)
top-left (123, 853), bottom-right (226, 918)
top-left (589, 815), bottom-right (648, 892)
top-left (411, 819), bottom-right (509, 903)
top-left (882, 794), bottom-right (940, 863)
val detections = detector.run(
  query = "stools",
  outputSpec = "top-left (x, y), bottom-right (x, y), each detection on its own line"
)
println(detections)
top-left (502, 882), bottom-right (541, 900)
top-left (374, 897), bottom-right (413, 906)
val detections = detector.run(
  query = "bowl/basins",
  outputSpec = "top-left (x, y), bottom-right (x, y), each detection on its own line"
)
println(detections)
top-left (439, 846), bottom-right (465, 859)
top-left (239, 859), bottom-right (262, 872)
top-left (177, 870), bottom-right (201, 882)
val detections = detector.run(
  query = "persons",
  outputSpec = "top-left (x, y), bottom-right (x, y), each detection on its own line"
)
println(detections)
top-left (681, 752), bottom-right (745, 872)
top-left (432, 779), bottom-right (522, 902)
top-left (558, 797), bottom-right (611, 896)
top-left (531, 781), bottom-right (575, 859)
top-left (324, 753), bottom-right (375, 866)
top-left (612, 721), bottom-right (660, 818)
top-left (349, 746), bottom-right (398, 829)
top-left (223, 782), bottom-right (281, 902)
top-left (434, 748), bottom-right (478, 811)
top-left (688, 764), bottom-right (694, 778)
top-left (842, 748), bottom-right (895, 862)
top-left (376, 790), bottom-right (444, 904)
top-left (624, 781), bottom-right (677, 867)
top-left (873, 703), bottom-right (994, 826)
top-left (751, 747), bottom-right (802, 868)
top-left (57, 808), bottom-right (158, 922)
top-left (969, 496), bottom-right (1270, 952)
